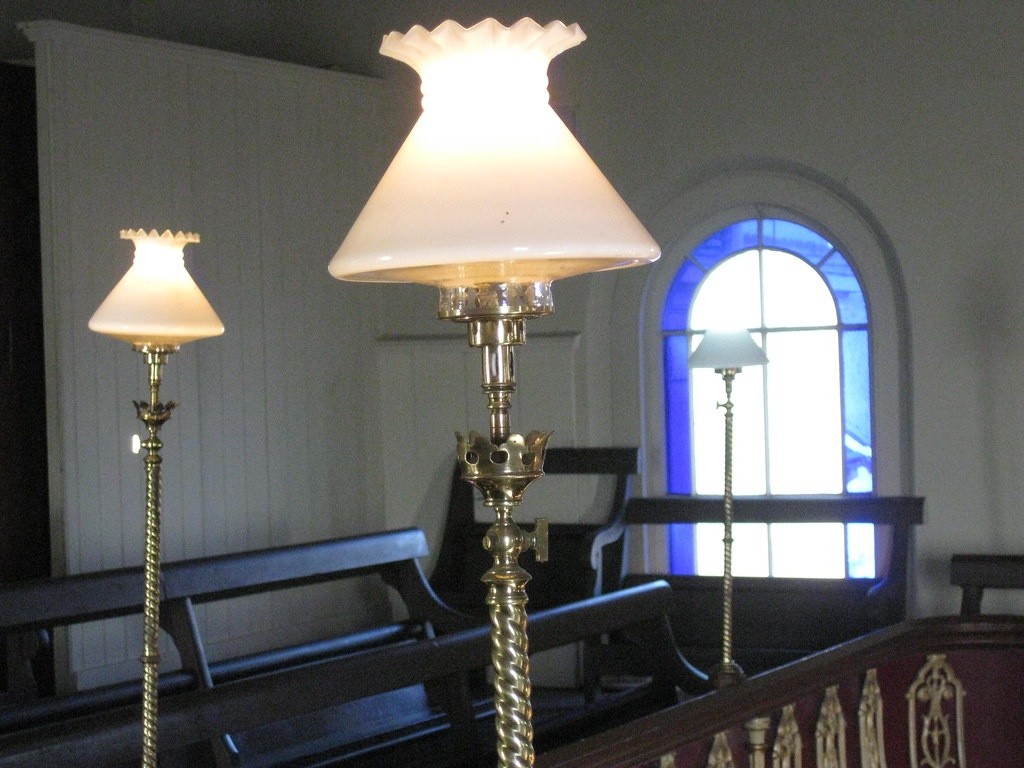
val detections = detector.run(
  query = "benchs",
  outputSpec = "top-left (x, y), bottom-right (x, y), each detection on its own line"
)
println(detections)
top-left (0, 527), bottom-right (711, 768)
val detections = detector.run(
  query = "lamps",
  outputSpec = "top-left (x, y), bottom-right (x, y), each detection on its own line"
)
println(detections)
top-left (326, 17), bottom-right (660, 768)
top-left (690, 329), bottom-right (771, 685)
top-left (88, 228), bottom-right (226, 768)
